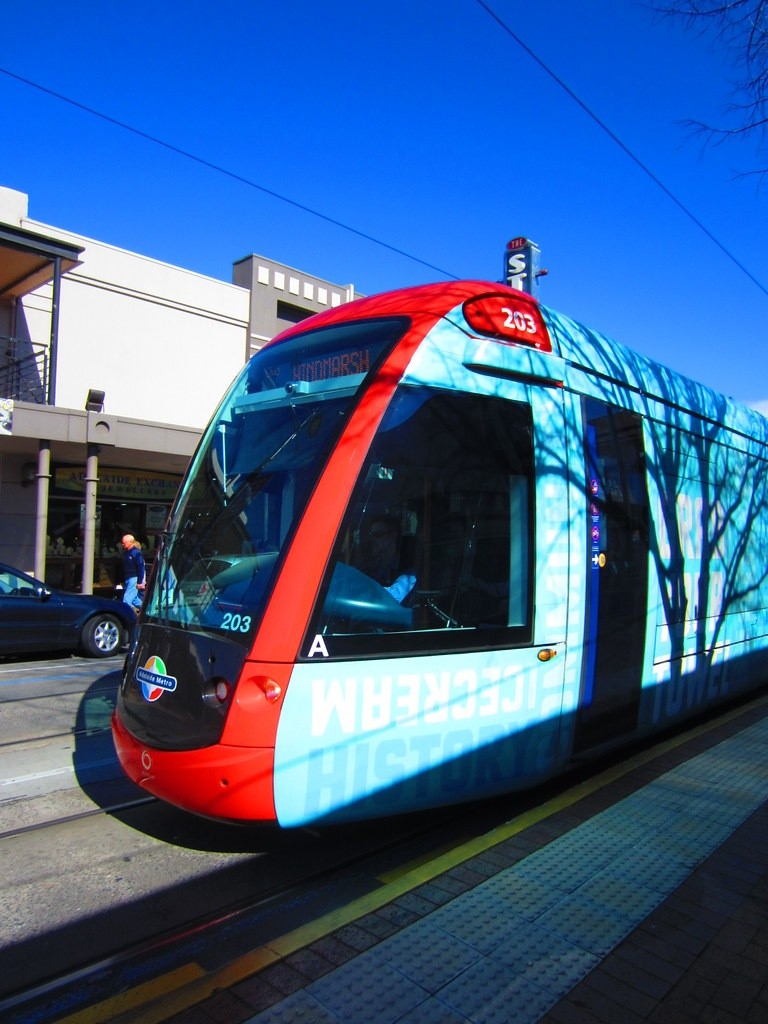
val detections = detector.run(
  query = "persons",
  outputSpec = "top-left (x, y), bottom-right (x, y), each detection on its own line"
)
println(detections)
top-left (119, 535), bottom-right (146, 608)
top-left (344, 514), bottom-right (403, 585)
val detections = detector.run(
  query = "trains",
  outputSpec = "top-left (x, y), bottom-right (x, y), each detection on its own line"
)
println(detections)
top-left (112, 283), bottom-right (768, 833)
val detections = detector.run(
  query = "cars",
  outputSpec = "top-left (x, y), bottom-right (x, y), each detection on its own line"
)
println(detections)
top-left (0, 563), bottom-right (136, 658)
